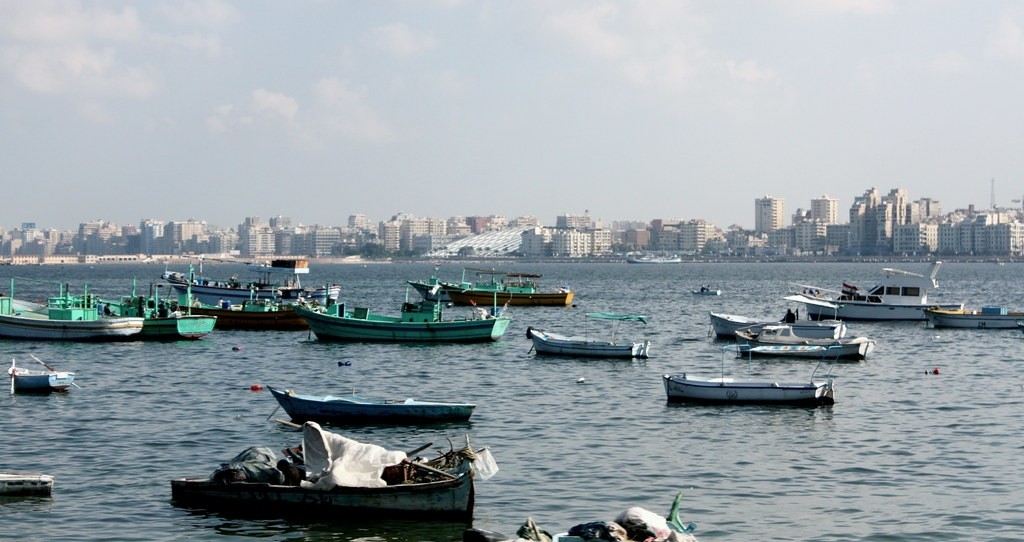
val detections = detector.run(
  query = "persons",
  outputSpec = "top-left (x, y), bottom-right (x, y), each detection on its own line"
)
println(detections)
top-left (701, 285), bottom-right (710, 292)
top-left (803, 288), bottom-right (819, 295)
top-left (780, 309), bottom-right (796, 323)
top-left (104, 303), bottom-right (112, 316)
top-left (219, 298), bottom-right (223, 306)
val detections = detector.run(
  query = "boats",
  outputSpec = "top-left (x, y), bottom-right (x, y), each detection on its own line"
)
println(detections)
top-left (733, 322), bottom-right (876, 360)
top-left (626, 252), bottom-right (683, 263)
top-left (7, 353), bottom-right (76, 390)
top-left (437, 270), bottom-right (574, 308)
top-left (161, 267), bottom-right (343, 309)
top-left (157, 273), bottom-right (326, 329)
top-left (266, 382), bottom-right (479, 426)
top-left (0, 472), bottom-right (54, 494)
top-left (706, 294), bottom-right (846, 340)
top-left (58, 273), bottom-right (219, 341)
top-left (662, 344), bottom-right (840, 407)
top-left (249, 262), bottom-right (275, 274)
top-left (168, 434), bottom-right (499, 522)
top-left (405, 276), bottom-right (468, 302)
top-left (0, 276), bottom-right (147, 340)
top-left (288, 285), bottom-right (514, 342)
top-left (526, 310), bottom-right (654, 361)
top-left (1017, 320), bottom-right (1024, 331)
top-left (787, 261), bottom-right (944, 324)
top-left (922, 302), bottom-right (1024, 329)
top-left (688, 289), bottom-right (723, 296)
